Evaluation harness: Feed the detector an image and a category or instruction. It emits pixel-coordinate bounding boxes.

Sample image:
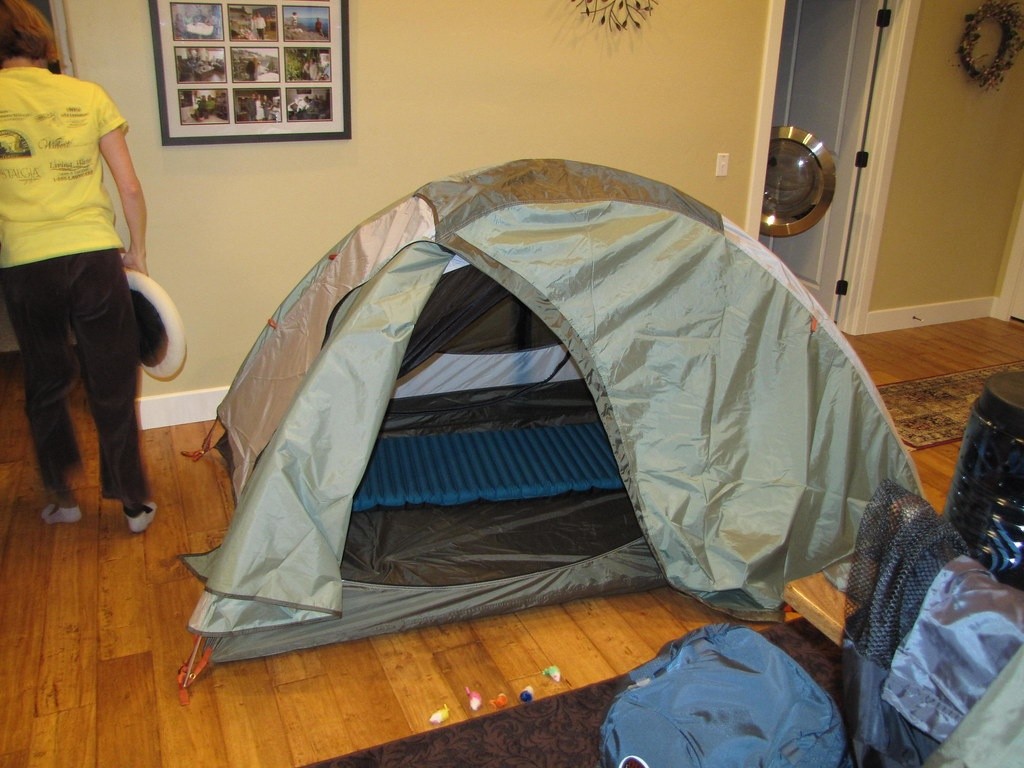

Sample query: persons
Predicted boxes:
[0,0,157,533]
[172,3,331,126]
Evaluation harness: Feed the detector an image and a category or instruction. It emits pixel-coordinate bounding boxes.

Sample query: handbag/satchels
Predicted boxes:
[600,622,846,768]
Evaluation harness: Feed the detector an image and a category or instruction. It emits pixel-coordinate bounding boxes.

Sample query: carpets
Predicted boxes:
[295,615,843,768]
[877,359,1024,452]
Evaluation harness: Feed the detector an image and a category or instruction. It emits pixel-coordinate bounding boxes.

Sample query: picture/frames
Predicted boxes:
[148,0,351,146]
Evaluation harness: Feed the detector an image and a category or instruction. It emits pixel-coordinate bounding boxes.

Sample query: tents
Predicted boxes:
[178,154,926,688]
